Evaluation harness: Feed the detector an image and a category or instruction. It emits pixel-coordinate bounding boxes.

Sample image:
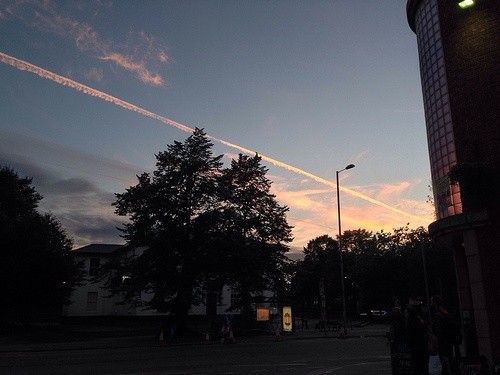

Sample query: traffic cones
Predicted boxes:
[219,323,237,345]
[272,324,284,342]
[158,328,167,347]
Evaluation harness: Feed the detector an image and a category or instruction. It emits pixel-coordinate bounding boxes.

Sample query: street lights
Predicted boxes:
[419,234,434,322]
[336,164,355,336]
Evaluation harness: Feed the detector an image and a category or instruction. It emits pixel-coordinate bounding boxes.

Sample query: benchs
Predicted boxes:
[314,322,342,332]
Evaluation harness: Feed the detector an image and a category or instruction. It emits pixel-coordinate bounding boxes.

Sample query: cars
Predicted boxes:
[359,308,390,322]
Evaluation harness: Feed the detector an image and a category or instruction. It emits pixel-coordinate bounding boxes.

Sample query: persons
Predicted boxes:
[446,294,463,365]
[220,308,237,344]
[438,191,451,211]
[430,294,454,375]
[383,306,432,375]
